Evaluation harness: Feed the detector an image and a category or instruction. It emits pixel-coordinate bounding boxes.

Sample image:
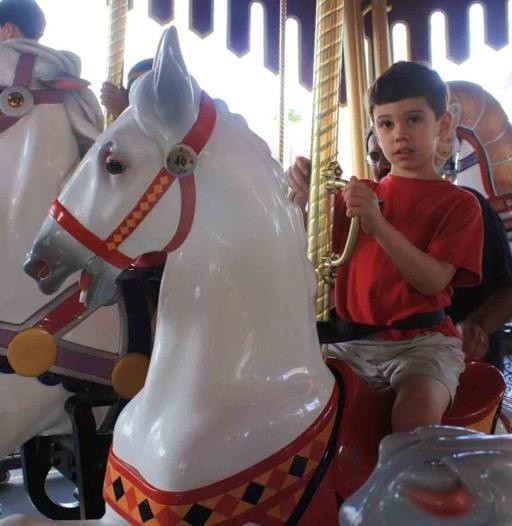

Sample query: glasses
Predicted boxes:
[366,152,381,165]
